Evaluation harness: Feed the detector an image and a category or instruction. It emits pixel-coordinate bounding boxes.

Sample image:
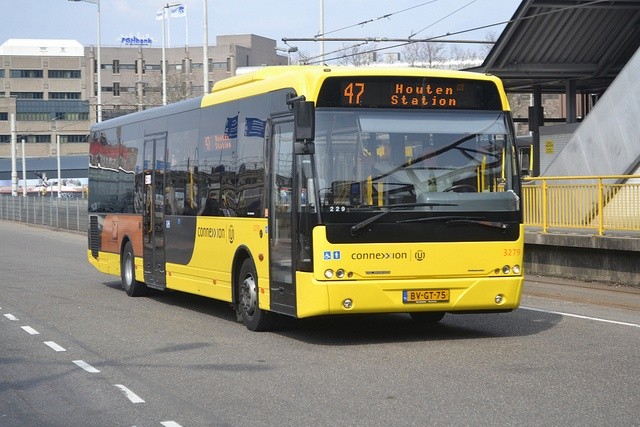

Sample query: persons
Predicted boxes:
[357,139,493,199]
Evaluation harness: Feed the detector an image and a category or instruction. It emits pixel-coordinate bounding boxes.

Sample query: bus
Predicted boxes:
[86,66,525,333]
